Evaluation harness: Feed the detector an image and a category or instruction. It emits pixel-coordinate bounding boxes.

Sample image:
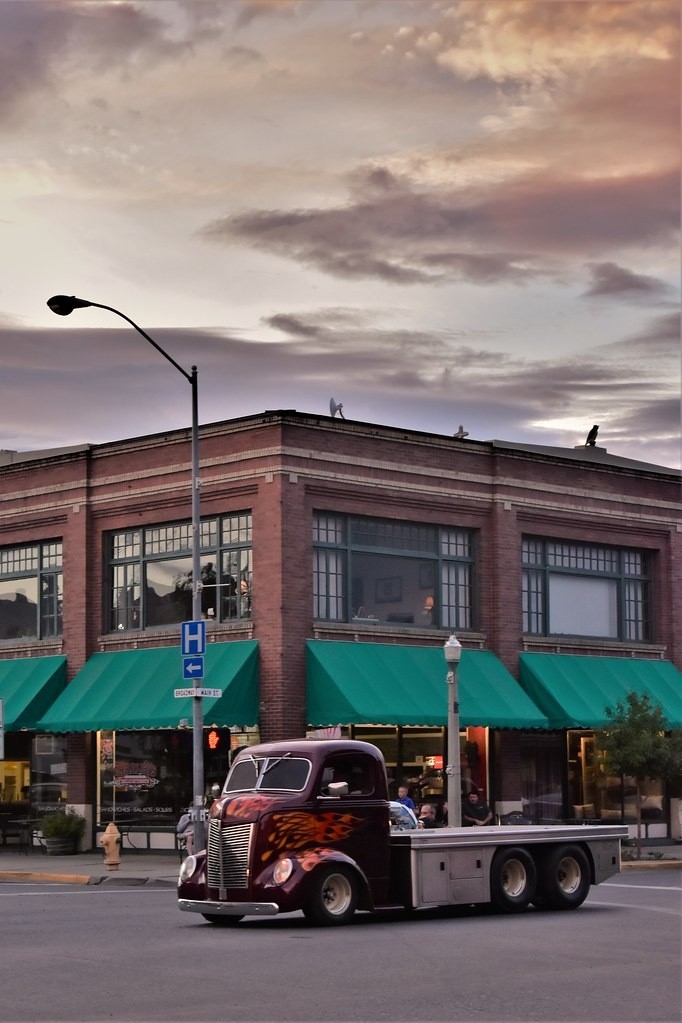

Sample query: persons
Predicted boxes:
[321,765,361,796]
[463,792,492,825]
[177,801,208,856]
[418,804,443,829]
[395,786,416,816]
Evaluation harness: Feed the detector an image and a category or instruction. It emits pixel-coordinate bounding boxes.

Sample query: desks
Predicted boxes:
[10,818,43,857]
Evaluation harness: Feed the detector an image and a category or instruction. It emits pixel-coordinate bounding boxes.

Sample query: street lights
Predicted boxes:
[443,634,463,826]
[47,293,206,854]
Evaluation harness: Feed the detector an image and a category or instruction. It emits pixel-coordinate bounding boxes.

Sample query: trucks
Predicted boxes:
[176,739,629,927]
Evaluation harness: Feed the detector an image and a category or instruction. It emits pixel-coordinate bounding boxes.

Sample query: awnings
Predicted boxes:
[518,651,682,730]
[37,639,260,729]
[305,639,549,728]
[0,654,67,732]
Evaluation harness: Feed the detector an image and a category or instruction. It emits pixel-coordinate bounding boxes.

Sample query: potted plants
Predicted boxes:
[40,808,86,856]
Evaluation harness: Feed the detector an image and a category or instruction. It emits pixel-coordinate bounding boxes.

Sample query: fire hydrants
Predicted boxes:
[100,822,122,871]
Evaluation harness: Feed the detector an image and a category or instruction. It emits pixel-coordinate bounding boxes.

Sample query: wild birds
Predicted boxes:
[585,425,599,446]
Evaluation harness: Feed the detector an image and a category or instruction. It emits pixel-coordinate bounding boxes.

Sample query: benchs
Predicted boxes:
[0,813,24,850]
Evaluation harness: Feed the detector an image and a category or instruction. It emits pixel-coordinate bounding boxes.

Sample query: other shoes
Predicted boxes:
[176,834,184,839]
